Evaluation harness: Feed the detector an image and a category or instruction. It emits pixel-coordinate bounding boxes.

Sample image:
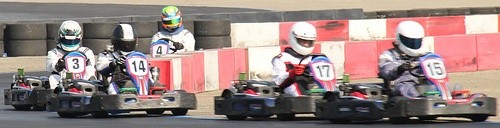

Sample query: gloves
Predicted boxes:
[288,66,305,78]
[399,63,413,75]
[109,55,126,69]
[55,58,66,71]
[173,42,184,51]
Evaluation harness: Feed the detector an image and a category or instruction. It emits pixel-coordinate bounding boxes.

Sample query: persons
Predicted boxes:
[96,24,170,103]
[149,5,195,56]
[45,20,96,98]
[271,21,370,113]
[377,21,480,108]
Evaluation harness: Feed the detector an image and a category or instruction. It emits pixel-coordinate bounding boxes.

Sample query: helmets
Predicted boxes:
[56,20,83,52]
[111,23,138,54]
[161,6,183,31]
[289,21,316,56]
[395,21,425,56]
